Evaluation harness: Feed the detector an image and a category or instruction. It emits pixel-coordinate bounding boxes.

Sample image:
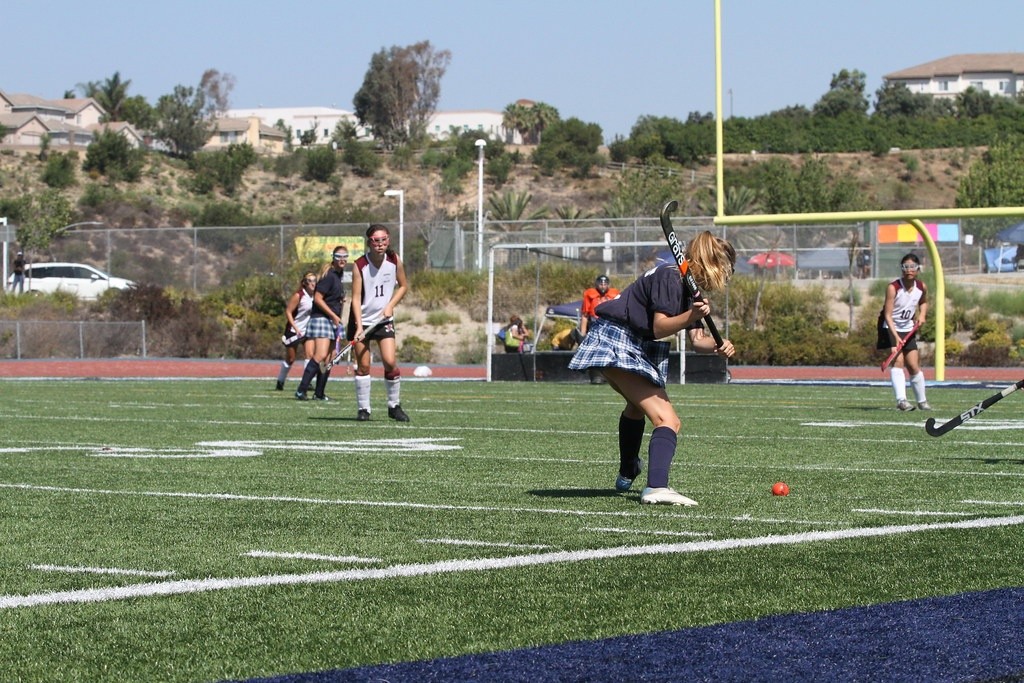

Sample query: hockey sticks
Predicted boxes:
[335,321,340,365]
[318,314,386,375]
[925,379,1024,438]
[280,331,306,346]
[661,200,724,348]
[881,321,919,372]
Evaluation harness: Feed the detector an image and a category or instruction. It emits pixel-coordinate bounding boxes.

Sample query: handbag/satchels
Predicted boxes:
[506,327,520,347]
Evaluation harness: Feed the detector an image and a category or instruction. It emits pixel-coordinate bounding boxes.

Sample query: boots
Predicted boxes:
[387,403,409,422]
[354,408,372,421]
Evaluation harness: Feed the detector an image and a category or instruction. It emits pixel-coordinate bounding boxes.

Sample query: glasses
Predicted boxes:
[369,236,389,246]
[304,278,317,284]
[334,253,349,261]
[900,263,920,272]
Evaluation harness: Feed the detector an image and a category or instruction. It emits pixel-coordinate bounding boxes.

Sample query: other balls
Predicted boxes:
[773,482,789,497]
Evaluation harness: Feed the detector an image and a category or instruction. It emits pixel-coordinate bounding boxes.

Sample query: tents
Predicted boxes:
[988,220,1024,276]
[531,300,585,354]
[747,251,796,281]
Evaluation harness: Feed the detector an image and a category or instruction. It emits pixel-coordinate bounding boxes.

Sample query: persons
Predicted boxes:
[551,328,579,351]
[276,262,338,391]
[567,231,736,507]
[581,275,622,385]
[10,251,26,293]
[295,246,349,402]
[347,225,410,422]
[856,242,872,280]
[877,254,932,411]
[504,315,529,352]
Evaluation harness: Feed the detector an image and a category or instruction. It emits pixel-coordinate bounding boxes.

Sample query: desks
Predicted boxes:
[491,350,728,384]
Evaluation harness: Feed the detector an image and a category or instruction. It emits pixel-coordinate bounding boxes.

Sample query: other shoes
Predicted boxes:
[294,390,311,401]
[276,380,285,391]
[313,394,332,401]
[307,383,316,391]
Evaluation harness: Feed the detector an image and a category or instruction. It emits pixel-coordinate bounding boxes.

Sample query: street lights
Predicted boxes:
[475,139,487,272]
[384,189,403,260]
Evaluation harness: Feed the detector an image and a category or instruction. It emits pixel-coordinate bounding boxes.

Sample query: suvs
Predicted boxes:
[7,262,137,302]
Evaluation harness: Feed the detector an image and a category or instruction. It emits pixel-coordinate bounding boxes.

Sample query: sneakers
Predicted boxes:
[616,457,643,492]
[640,486,698,507]
[917,401,932,411]
[896,400,915,412]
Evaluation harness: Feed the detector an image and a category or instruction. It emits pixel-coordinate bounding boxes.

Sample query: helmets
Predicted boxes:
[596,275,609,294]
